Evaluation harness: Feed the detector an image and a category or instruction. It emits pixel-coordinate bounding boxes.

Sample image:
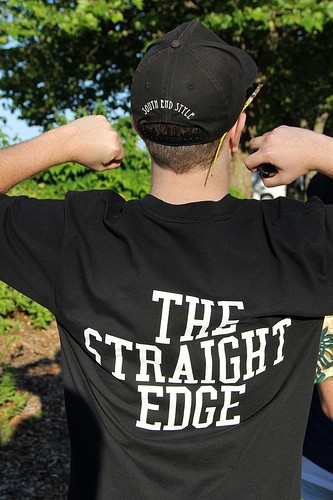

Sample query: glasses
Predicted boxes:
[204,83,265,188]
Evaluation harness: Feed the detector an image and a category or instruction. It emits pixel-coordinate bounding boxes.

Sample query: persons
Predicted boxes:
[302,315,333,499]
[0,20,333,500]
[251,127,307,202]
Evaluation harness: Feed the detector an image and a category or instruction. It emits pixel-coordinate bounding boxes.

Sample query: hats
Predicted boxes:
[131,20,257,146]
